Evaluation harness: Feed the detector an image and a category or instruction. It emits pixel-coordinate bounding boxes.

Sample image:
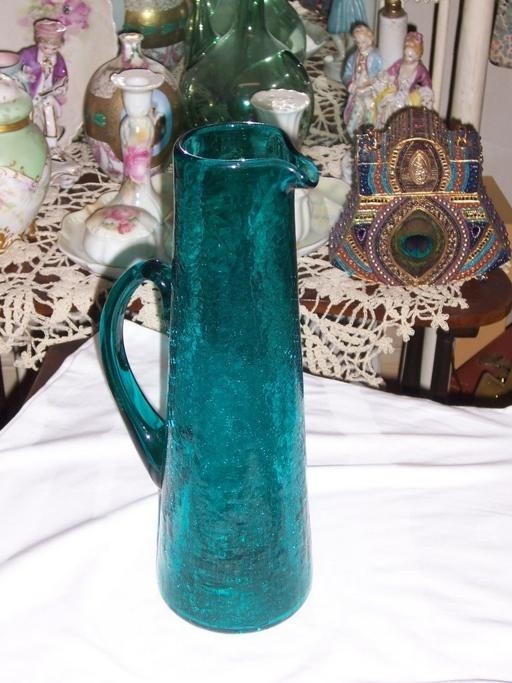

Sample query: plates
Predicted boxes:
[56,168,355,279]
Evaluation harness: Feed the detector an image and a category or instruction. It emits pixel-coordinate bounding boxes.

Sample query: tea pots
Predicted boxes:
[97,123,322,634]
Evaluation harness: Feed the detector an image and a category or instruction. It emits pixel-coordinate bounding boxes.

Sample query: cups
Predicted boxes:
[163,208,176,259]
